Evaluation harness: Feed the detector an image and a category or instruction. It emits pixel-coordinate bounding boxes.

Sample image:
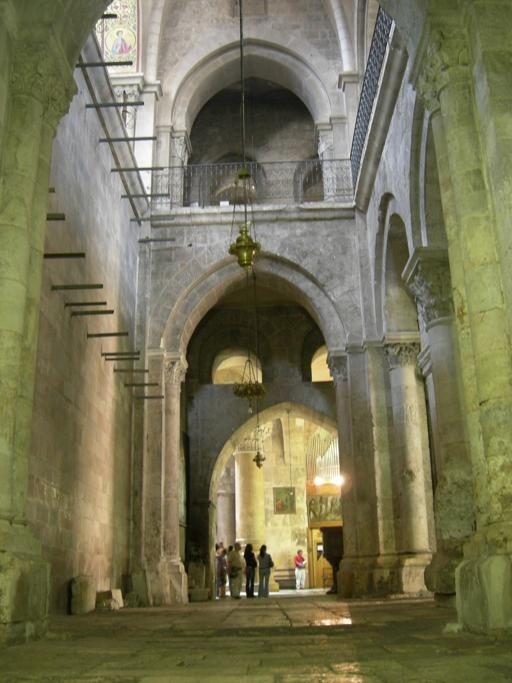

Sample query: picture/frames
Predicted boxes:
[272,485,296,515]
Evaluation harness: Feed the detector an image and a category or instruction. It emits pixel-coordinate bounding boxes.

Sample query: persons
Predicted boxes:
[295,549,306,590]
[244,544,257,598]
[256,544,271,598]
[216,541,243,599]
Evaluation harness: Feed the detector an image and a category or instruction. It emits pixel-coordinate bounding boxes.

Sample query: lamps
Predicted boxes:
[210,264,267,470]
[225,1,261,267]
